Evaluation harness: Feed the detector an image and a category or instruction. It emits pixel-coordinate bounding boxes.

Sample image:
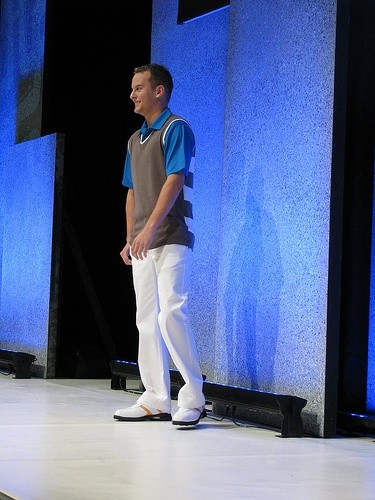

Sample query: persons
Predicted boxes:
[112,61,208,428]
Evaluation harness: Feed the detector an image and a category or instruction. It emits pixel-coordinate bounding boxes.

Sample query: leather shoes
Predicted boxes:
[113,402,172,424]
[171,403,207,427]
